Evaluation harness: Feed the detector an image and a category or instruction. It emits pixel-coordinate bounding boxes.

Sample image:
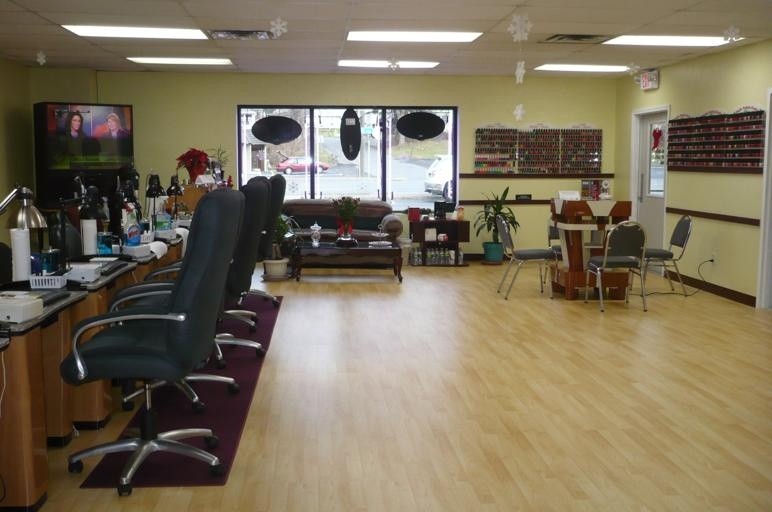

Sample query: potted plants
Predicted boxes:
[264,217,291,280]
[474,189,518,262]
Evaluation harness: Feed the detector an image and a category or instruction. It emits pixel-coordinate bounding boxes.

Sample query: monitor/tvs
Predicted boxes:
[33,102,135,174]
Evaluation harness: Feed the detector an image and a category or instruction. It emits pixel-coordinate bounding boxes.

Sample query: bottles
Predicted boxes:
[474,124,600,174]
[41,246,62,275]
[426,248,455,264]
[458,247,464,264]
[456,206,464,220]
[667,112,764,167]
[410,248,422,265]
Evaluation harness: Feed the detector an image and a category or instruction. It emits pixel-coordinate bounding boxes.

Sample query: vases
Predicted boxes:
[336,216,353,239]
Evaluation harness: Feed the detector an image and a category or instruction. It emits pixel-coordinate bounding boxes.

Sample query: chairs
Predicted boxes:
[496,209,689,313]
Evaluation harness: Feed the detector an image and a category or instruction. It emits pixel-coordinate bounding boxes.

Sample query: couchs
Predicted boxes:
[283,200,403,240]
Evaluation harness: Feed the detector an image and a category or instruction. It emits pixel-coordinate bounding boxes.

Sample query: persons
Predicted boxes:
[65,112,86,139]
[100,113,129,139]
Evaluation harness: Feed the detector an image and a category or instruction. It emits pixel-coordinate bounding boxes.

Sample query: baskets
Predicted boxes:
[28,270,71,289]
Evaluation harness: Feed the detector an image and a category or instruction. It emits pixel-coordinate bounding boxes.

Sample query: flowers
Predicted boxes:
[176,149,208,181]
[332,196,361,215]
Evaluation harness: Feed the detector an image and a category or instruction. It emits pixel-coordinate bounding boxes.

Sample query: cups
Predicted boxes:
[156,214,171,230]
[438,234,448,242]
[98,232,113,254]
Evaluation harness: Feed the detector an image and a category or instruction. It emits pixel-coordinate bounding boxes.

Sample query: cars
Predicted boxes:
[277,156,330,175]
[424,154,449,199]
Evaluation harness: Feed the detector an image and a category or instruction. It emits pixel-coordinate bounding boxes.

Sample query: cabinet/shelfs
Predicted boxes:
[669,110,764,173]
[459,127,614,178]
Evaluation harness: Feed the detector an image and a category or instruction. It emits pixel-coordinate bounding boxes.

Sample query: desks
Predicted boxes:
[411,219,470,266]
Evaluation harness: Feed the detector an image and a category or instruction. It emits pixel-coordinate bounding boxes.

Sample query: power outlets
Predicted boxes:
[709,255,719,265]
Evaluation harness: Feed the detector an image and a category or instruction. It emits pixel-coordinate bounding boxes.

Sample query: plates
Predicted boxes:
[369,241,392,247]
[372,233,390,237]
